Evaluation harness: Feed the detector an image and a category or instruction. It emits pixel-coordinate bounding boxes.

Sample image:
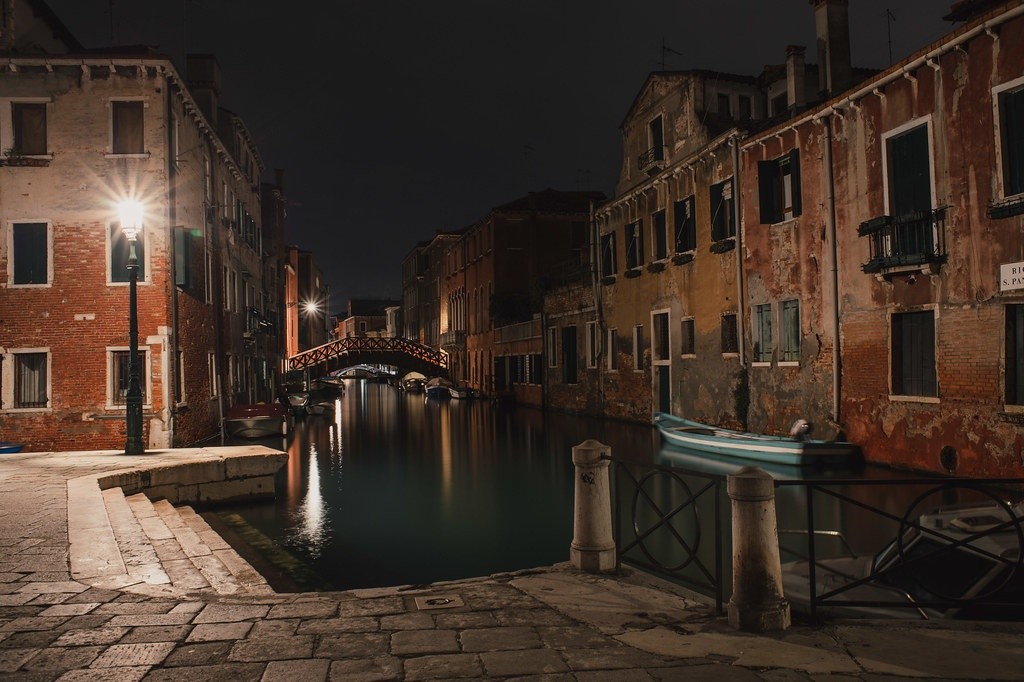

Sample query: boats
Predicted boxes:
[224,391,310,440]
[658,443,857,499]
[399,371,474,399]
[653,412,855,465]
[777,499,1024,620]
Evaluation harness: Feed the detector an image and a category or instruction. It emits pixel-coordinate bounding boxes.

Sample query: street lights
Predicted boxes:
[115,196,147,453]
